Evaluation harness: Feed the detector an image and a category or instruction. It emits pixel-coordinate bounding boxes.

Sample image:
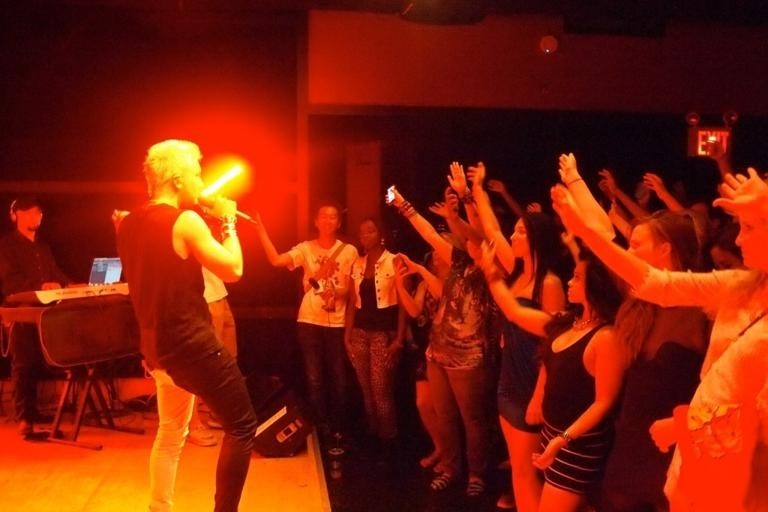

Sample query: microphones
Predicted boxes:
[308,276,336,311]
[195,192,248,221]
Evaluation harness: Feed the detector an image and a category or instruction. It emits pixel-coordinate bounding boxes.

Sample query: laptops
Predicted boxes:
[86,255,123,283]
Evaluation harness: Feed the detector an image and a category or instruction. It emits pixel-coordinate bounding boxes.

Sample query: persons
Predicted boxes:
[255,139,767,511]
[1,195,73,440]
[115,140,256,512]
[187,267,238,446]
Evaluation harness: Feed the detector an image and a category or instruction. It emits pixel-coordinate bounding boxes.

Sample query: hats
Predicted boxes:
[9,194,48,211]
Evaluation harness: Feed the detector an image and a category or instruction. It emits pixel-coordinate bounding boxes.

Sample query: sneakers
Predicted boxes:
[359,428,487,497]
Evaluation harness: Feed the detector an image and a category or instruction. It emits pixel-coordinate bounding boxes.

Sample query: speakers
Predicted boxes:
[243,367,313,459]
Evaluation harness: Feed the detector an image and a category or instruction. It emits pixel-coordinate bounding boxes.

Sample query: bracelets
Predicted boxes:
[220,217,238,239]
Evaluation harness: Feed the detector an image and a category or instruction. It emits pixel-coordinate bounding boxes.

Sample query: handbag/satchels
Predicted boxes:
[252,363,310,457]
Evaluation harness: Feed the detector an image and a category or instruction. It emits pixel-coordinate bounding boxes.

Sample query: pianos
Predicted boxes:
[5,282,129,305]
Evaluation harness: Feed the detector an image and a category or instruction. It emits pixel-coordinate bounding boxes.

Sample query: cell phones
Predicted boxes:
[388,185,395,204]
